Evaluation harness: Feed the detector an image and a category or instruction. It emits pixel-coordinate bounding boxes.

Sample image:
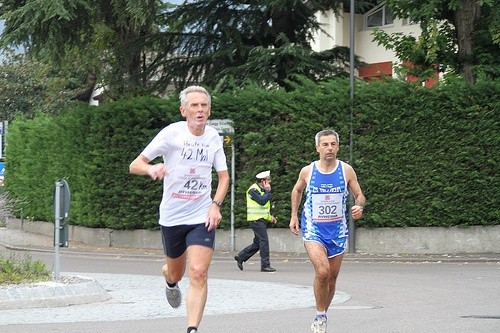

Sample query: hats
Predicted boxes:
[255,170,272,180]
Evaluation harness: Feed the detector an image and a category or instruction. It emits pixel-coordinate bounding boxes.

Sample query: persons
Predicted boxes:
[235,170,276,272]
[289,129,366,333]
[129,84,230,333]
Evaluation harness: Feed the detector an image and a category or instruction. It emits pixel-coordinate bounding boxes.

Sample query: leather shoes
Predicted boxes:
[261,267,276,272]
[234,255,243,270]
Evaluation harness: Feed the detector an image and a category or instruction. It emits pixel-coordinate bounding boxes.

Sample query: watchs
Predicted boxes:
[212,199,222,207]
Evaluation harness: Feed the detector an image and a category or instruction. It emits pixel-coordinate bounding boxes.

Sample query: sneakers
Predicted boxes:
[166,282,182,308]
[311,314,328,333]
